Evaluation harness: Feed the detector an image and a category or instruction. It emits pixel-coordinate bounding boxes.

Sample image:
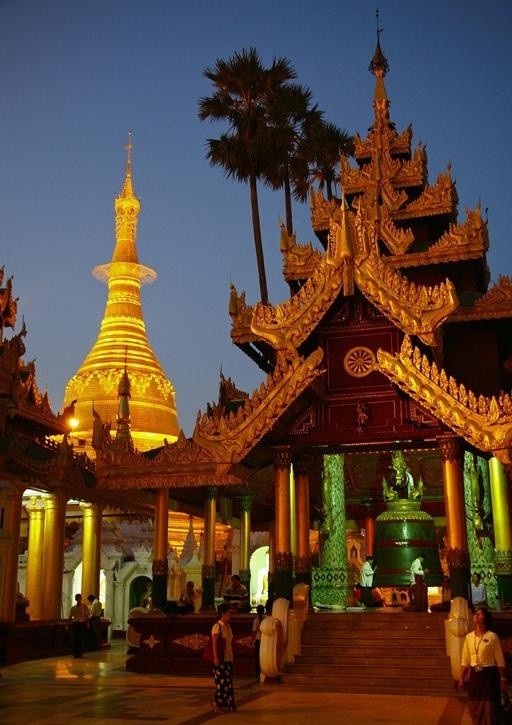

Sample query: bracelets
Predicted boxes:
[501,677,508,682]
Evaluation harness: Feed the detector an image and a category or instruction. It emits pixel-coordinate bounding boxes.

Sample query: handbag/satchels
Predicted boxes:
[202,622,225,665]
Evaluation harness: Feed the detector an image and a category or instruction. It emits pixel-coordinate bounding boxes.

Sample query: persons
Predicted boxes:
[456,608,509,725]
[66,594,89,654]
[222,574,252,613]
[15,593,30,624]
[472,572,488,611]
[251,605,268,680]
[357,556,379,608]
[88,592,102,648]
[402,574,429,612]
[172,582,199,616]
[409,553,429,600]
[211,603,246,714]
